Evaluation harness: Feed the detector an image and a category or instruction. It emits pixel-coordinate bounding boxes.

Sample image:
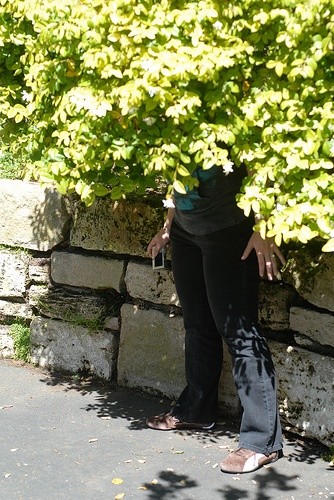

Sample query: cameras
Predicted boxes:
[152,246,164,269]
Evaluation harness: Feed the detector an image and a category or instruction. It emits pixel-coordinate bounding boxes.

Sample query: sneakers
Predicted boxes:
[146,409,216,431]
[220,447,279,473]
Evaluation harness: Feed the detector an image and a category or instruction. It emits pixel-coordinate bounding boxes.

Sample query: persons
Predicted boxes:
[146,140,289,473]
